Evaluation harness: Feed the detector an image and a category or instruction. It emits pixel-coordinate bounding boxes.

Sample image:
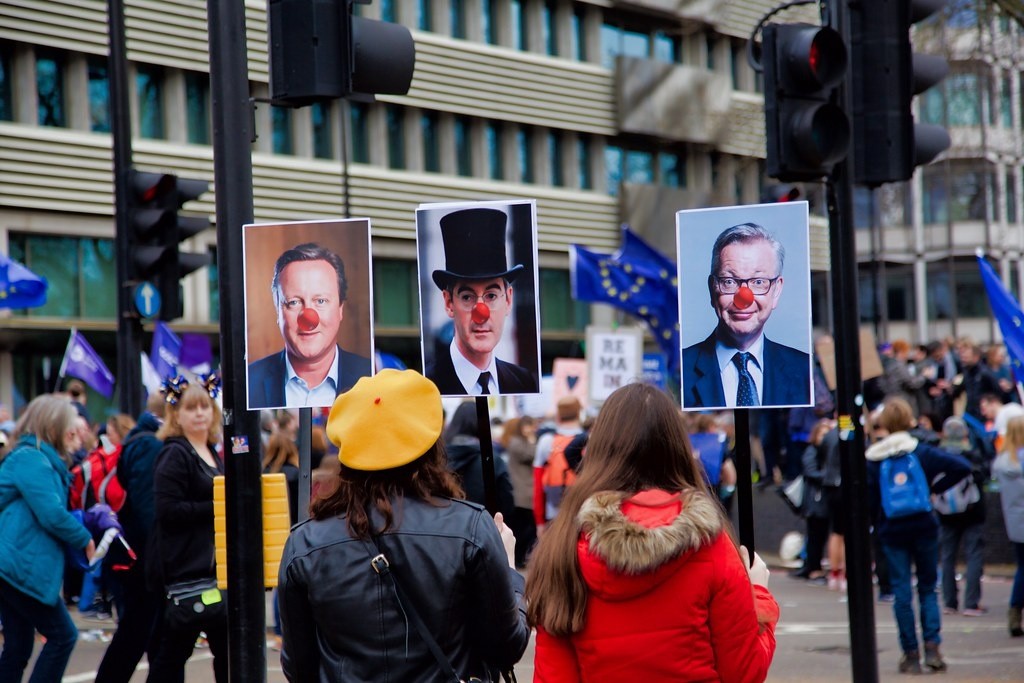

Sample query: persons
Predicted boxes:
[446,397,595,572]
[866,336,1024,676]
[277,369,532,683]
[526,382,781,683]
[682,409,846,593]
[249,242,372,408]
[426,208,536,395]
[0,382,226,683]
[260,410,339,650]
[683,222,810,408]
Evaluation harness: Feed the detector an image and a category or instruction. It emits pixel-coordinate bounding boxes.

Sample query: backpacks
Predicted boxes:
[876,442,934,516]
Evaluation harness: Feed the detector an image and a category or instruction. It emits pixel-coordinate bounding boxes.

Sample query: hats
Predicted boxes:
[326,367,445,468]
[943,417,969,438]
[557,398,580,418]
[432,206,524,290]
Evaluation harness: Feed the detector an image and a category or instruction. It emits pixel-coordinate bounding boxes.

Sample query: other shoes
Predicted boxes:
[876,594,895,605]
[828,569,846,590]
[964,606,990,616]
[943,605,958,616]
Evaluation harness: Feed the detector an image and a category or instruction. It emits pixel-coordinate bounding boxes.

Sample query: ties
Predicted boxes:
[478,371,491,395]
[732,351,759,406]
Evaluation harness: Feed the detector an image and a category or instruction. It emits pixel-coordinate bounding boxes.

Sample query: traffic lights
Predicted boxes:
[266,1,415,105]
[129,171,212,321]
[823,0,953,191]
[763,21,850,182]
[766,187,798,208]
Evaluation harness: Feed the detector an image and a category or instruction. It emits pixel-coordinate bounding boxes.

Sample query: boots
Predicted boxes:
[1007,608,1023,636]
[925,641,946,671]
[899,650,919,673]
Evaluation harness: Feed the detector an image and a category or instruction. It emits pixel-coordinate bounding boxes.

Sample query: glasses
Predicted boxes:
[714,273,779,295]
[450,289,508,311]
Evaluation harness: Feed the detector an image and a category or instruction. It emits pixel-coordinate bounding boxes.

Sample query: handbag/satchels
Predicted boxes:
[461,657,517,683]
[783,475,805,515]
[169,578,221,627]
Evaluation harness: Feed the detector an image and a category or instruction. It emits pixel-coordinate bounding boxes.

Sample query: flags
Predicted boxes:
[64,335,115,401]
[977,256,1024,403]
[572,227,679,376]
[0,253,49,309]
